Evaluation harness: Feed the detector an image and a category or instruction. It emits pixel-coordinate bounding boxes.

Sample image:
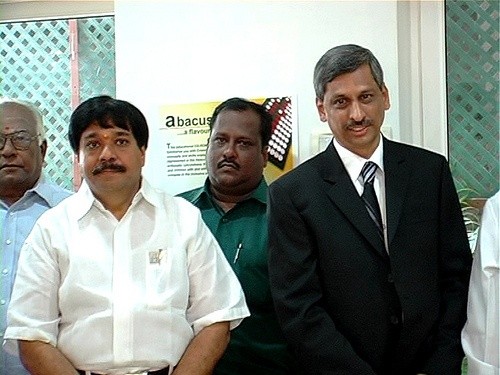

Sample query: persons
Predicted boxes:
[265,43,474,375]
[166,93,276,375]
[0,101,76,373]
[6,93,251,375]
[461,191,499,375]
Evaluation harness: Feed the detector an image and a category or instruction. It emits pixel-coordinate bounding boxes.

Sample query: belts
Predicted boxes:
[75,365,169,375]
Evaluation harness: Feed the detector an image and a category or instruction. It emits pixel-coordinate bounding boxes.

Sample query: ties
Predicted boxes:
[361,161,385,247]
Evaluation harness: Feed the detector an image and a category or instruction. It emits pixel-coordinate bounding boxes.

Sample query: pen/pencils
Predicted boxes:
[231,241,244,265]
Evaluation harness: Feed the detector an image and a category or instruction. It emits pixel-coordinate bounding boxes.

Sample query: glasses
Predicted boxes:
[0,129,38,151]
